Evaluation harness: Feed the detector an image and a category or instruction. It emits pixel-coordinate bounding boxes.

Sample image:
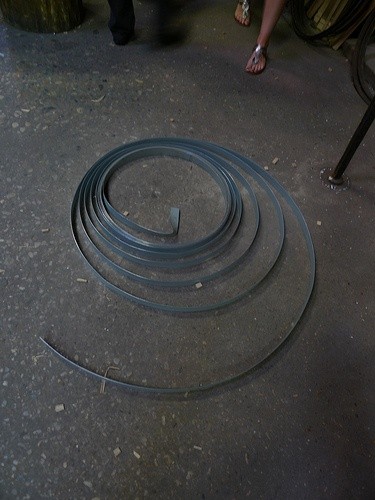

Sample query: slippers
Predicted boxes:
[235,0,251,26]
[249,43,266,74]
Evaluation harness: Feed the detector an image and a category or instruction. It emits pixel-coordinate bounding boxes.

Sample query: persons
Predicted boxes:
[107,0,189,48]
[234,0,284,75]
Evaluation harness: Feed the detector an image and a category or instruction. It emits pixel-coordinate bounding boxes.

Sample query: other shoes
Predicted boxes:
[114,30,137,46]
[154,30,187,49]
